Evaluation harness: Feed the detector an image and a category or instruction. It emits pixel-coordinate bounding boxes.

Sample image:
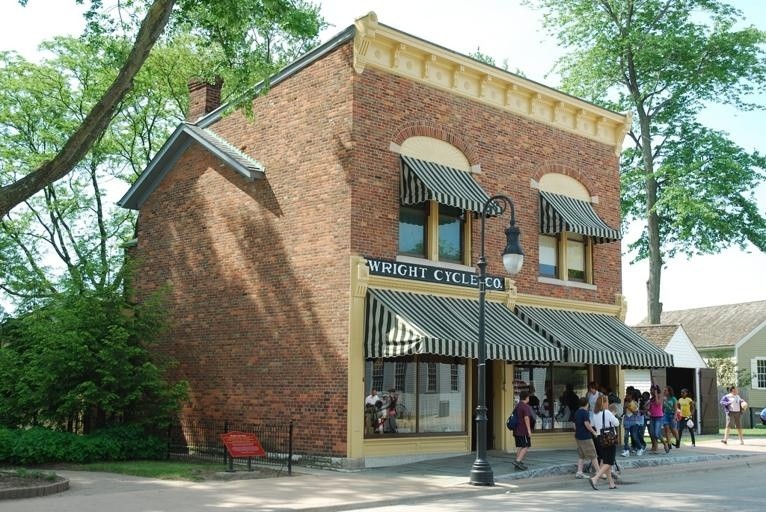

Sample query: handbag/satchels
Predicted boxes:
[506,412,520,431]
[600,427,619,448]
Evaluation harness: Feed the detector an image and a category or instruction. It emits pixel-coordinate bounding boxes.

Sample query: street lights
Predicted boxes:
[469,193,525,488]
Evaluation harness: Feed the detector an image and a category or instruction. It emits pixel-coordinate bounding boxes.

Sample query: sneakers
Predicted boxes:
[620,446,648,458]
[609,480,617,488]
[589,477,599,490]
[512,460,525,471]
[575,472,590,479]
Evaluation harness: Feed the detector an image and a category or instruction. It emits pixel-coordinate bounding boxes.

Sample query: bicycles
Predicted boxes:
[371,388,411,422]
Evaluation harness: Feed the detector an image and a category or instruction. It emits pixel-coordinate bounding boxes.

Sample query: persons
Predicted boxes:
[527,383,580,430]
[720,384,747,444]
[571,380,696,491]
[364,385,399,433]
[512,390,535,471]
